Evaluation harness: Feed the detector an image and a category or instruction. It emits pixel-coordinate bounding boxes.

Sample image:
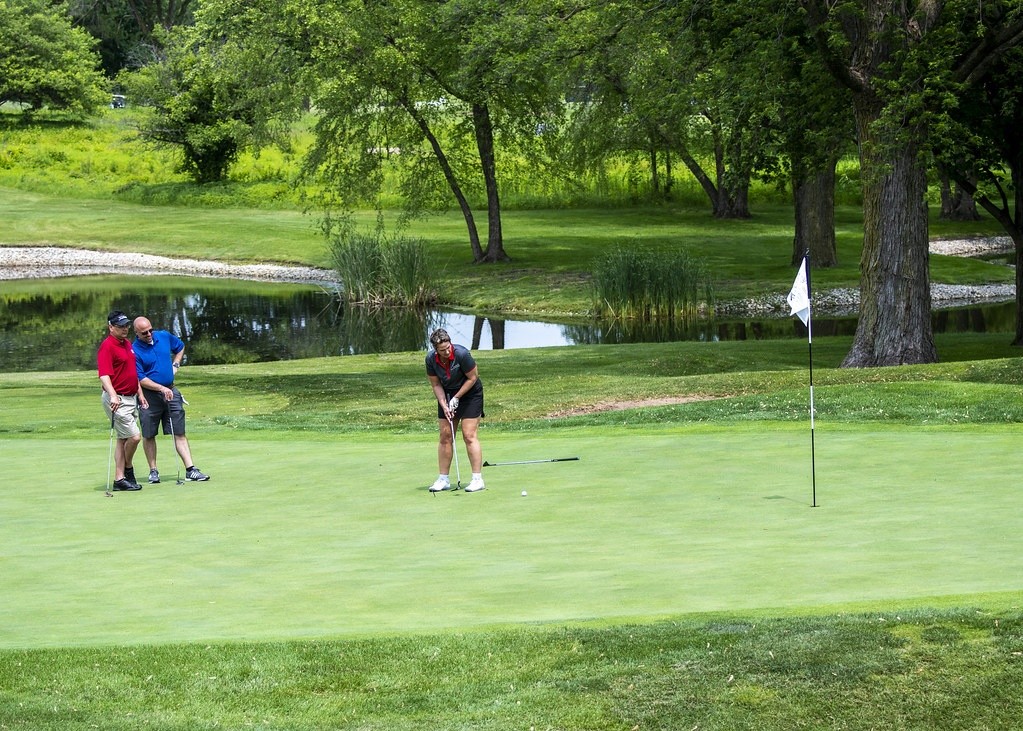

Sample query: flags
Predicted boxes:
[786,250,812,326]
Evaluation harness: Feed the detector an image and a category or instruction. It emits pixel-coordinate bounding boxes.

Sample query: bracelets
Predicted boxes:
[173,362,179,368]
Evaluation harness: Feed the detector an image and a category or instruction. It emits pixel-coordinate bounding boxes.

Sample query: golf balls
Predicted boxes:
[521,490,527,497]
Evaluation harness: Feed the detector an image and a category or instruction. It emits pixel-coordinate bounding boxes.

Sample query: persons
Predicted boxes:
[99,311,210,490]
[425,328,486,493]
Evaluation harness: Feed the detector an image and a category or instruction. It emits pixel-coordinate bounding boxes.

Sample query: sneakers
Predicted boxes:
[429,478,450,492]
[124,466,136,484]
[185,467,210,481]
[113,478,142,491]
[465,478,485,492]
[148,470,160,483]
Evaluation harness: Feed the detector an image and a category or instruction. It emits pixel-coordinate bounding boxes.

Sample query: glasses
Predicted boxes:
[138,328,154,335]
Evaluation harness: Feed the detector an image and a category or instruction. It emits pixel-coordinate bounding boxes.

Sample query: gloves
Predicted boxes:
[449,397,459,411]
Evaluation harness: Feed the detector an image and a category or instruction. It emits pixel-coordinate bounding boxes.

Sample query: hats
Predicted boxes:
[108,313,133,326]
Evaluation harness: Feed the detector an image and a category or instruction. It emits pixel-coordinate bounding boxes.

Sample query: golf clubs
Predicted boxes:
[165,394,186,486]
[482,456,580,468]
[104,405,116,498]
[446,393,462,492]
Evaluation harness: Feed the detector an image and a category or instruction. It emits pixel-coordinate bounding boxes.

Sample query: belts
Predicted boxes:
[142,383,174,393]
[117,392,134,396]
